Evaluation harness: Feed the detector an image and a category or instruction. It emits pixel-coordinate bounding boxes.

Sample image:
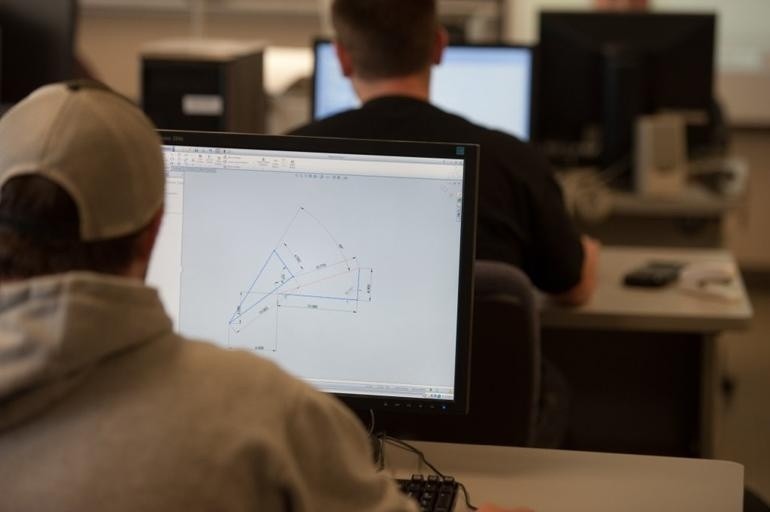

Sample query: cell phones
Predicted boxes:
[624,261,679,289]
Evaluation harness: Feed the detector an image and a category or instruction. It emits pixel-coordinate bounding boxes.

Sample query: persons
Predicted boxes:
[0,75,535,512]
[266,1,611,363]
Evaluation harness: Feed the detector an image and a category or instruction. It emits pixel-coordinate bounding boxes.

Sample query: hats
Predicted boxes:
[0,80,167,244]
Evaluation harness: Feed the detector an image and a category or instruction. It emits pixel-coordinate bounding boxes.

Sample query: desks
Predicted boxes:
[577,190,733,246]
[382,437,746,511]
[531,246,756,432]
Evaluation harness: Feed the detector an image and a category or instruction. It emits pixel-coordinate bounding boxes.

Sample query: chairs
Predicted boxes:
[384,257,544,443]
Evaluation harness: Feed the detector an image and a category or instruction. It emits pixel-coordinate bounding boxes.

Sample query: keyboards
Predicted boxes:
[385,470,459,512]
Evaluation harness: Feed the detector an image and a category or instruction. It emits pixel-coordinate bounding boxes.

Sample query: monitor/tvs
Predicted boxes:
[537,9,719,204]
[144,124,481,468]
[312,38,533,142]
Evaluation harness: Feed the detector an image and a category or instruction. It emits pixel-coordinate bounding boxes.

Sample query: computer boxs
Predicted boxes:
[136,41,265,131]
